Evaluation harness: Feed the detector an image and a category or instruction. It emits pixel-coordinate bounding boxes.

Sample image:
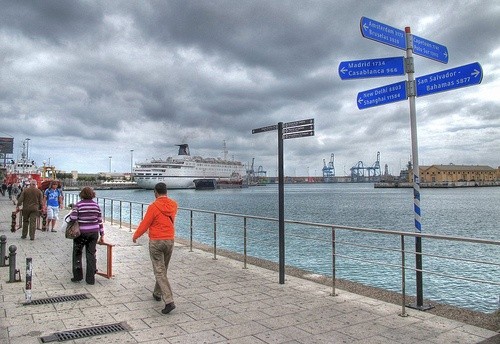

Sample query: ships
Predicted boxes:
[134,139,247,190]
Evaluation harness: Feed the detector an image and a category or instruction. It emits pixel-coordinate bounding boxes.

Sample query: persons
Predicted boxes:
[133,183,178,314]
[71,186,105,285]
[0,181,64,240]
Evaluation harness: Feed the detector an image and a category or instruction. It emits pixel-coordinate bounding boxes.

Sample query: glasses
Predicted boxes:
[54,184,57,185]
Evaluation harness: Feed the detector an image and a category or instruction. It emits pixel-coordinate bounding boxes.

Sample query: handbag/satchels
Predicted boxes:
[65,214,81,238]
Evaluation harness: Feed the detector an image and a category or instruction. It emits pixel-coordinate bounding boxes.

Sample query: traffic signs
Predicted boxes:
[357,79,408,109]
[338,56,407,80]
[360,16,408,50]
[282,118,314,139]
[251,125,278,134]
[413,34,449,65]
[415,62,483,97]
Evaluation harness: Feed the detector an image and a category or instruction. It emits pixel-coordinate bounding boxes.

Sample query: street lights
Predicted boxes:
[130,149,134,182]
[25,138,31,162]
[108,156,112,173]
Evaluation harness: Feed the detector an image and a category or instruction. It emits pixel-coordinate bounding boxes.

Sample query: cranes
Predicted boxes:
[350,151,382,177]
[321,153,335,178]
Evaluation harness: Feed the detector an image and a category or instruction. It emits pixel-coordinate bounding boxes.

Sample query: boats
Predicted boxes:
[101,179,137,190]
[5,140,63,197]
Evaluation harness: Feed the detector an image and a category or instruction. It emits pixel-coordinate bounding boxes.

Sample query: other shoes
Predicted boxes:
[161,303,176,314]
[71,278,81,283]
[21,236,26,239]
[30,237,34,240]
[153,293,161,301]
[51,229,57,232]
